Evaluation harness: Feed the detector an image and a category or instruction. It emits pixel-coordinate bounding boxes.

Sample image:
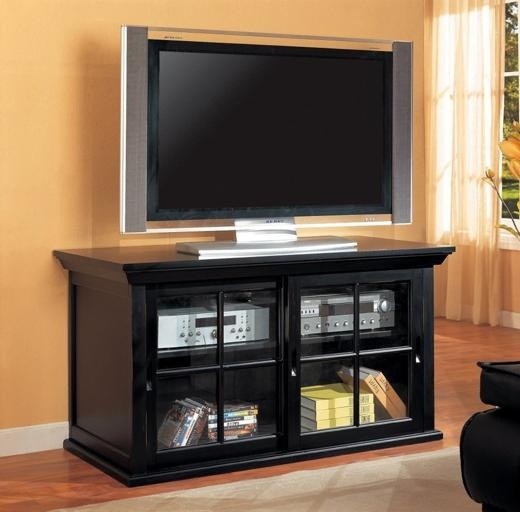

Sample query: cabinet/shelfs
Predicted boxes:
[51,234,456,488]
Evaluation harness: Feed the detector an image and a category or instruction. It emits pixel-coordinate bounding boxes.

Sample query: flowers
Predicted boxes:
[481,110,519,248]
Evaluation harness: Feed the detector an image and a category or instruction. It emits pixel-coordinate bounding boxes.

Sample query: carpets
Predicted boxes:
[69,446,483,511]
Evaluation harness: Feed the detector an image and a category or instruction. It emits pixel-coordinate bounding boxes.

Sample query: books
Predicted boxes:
[297,406,375,421]
[296,417,374,430]
[360,366,407,416]
[336,367,404,419]
[299,384,374,412]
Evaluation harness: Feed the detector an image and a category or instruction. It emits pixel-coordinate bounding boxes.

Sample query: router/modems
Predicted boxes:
[156,302,271,350]
[301,288,396,336]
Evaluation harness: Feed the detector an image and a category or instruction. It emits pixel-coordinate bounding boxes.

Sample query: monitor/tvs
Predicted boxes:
[119,23,414,261]
